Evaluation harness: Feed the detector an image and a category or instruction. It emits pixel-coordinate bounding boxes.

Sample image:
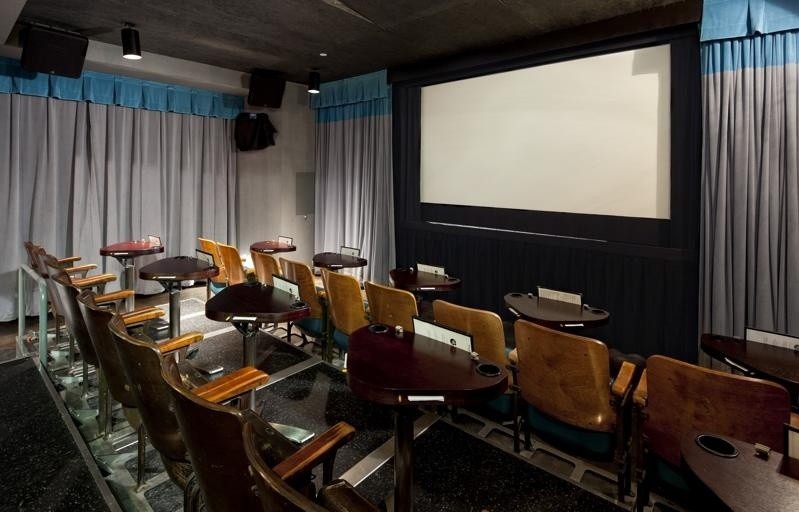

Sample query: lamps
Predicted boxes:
[302,66,324,96]
[121,19,144,61]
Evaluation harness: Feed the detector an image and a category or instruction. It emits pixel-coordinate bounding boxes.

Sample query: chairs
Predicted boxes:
[45,261,116,395]
[364,279,424,334]
[217,243,249,288]
[107,314,270,512]
[23,239,98,372]
[160,353,318,512]
[74,290,204,437]
[510,315,636,504]
[196,239,232,303]
[636,358,790,511]
[36,252,97,367]
[50,272,135,400]
[433,297,522,451]
[240,421,376,512]
[700,333,797,392]
[279,256,328,358]
[252,249,281,289]
[322,265,369,362]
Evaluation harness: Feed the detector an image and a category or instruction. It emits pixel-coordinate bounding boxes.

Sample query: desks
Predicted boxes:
[99,238,163,312]
[205,280,313,412]
[347,320,508,511]
[680,426,799,509]
[138,256,220,337]
[312,251,369,271]
[390,265,461,315]
[251,239,297,258]
[505,286,610,328]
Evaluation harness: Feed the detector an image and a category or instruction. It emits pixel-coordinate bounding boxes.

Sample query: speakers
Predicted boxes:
[248,69,286,108]
[20,24,90,79]
